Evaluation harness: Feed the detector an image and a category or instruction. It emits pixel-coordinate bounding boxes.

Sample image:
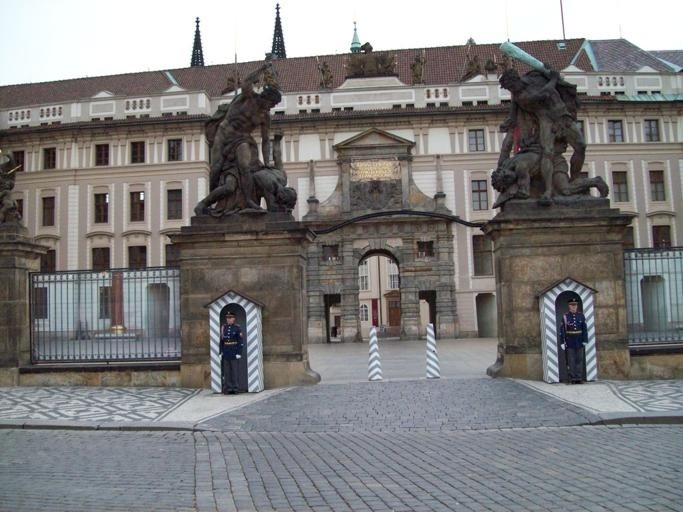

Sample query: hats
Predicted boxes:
[224,311,236,319]
[567,298,579,305]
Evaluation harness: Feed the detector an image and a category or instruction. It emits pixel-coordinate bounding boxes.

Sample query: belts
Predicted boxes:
[565,329,583,334]
[222,341,238,345]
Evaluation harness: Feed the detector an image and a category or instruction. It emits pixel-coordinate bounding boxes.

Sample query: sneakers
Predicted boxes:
[223,390,238,395]
[571,379,584,385]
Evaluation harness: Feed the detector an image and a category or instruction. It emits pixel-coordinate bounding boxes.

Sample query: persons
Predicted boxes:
[495,55,515,69]
[410,52,428,86]
[194,54,297,218]
[318,61,335,88]
[559,298,589,384]
[491,62,609,207]
[219,311,243,395]
[461,55,480,81]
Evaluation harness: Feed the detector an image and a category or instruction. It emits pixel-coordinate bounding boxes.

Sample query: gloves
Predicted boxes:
[236,354,242,360]
[218,352,224,361]
[581,342,588,347]
[560,343,566,351]
